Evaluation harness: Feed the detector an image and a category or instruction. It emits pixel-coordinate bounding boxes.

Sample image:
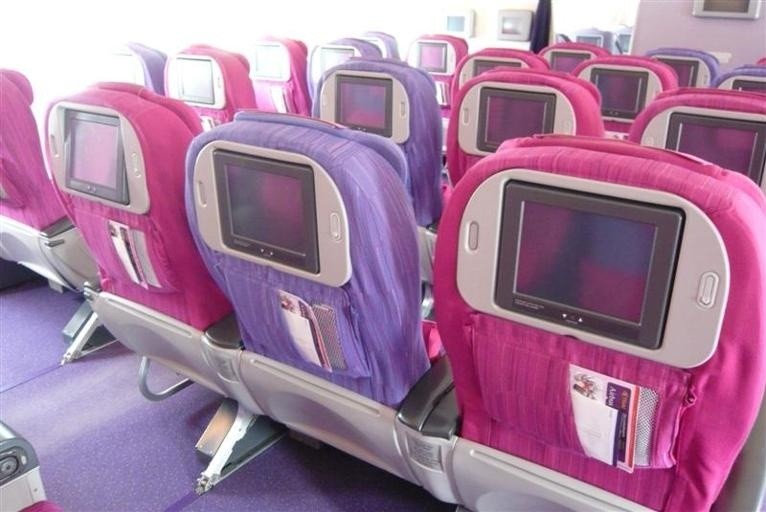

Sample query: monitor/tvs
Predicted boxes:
[170,56,217,107]
[211,150,322,275]
[334,73,394,138]
[61,108,132,204]
[317,46,354,74]
[656,57,700,90]
[500,15,523,33]
[476,87,556,152]
[416,41,447,73]
[79,117,86,121]
[665,108,766,188]
[252,42,290,79]
[446,15,463,31]
[732,80,766,93]
[495,181,683,349]
[473,59,522,74]
[577,35,597,44]
[551,51,590,76]
[590,67,649,125]
[91,56,146,84]
[617,33,628,49]
